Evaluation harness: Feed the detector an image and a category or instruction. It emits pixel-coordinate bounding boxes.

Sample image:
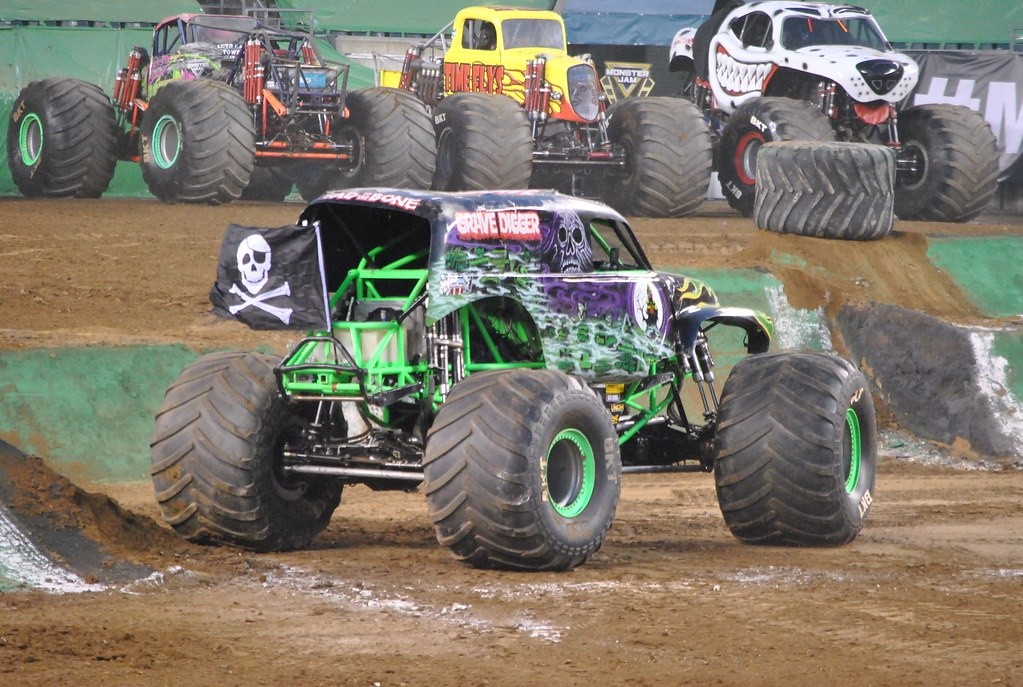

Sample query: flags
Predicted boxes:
[210,223,328,331]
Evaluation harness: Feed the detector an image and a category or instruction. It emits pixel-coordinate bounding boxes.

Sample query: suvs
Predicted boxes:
[147,186,879,572]
[666,0,998,225]
[5,12,437,206]
[397,4,713,219]
